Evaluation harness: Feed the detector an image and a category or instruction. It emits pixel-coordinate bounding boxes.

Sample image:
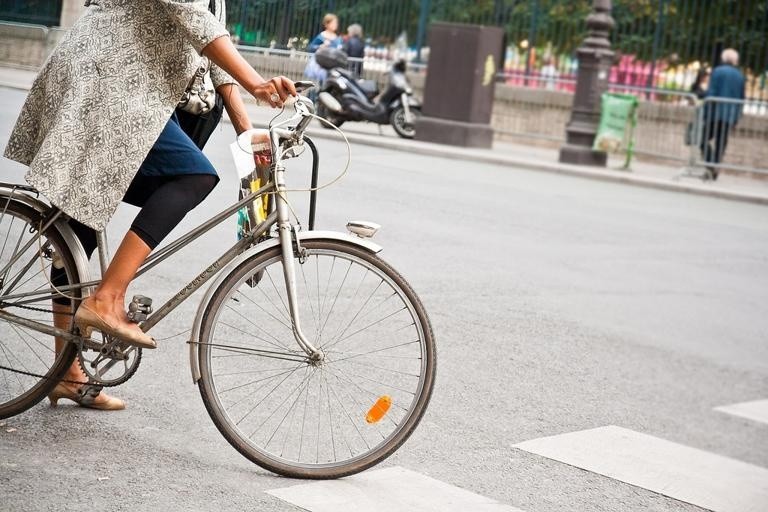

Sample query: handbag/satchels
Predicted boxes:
[230,128,279,288]
[179,54,218,116]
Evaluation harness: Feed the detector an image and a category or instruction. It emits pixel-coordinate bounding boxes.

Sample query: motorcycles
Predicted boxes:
[314,45,422,141]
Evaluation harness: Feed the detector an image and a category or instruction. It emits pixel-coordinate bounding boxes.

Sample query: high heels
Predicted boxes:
[74,300,157,349]
[47,380,127,411]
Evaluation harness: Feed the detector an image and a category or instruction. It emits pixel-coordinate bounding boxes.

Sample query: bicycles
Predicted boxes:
[0,80,435,477]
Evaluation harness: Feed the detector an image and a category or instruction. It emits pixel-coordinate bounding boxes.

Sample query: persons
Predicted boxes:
[539,55,559,92]
[302,13,343,116]
[694,49,746,182]
[3,1,298,409]
[684,62,712,147]
[338,23,364,78]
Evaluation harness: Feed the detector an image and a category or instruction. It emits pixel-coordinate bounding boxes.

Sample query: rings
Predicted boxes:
[271,93,279,103]
